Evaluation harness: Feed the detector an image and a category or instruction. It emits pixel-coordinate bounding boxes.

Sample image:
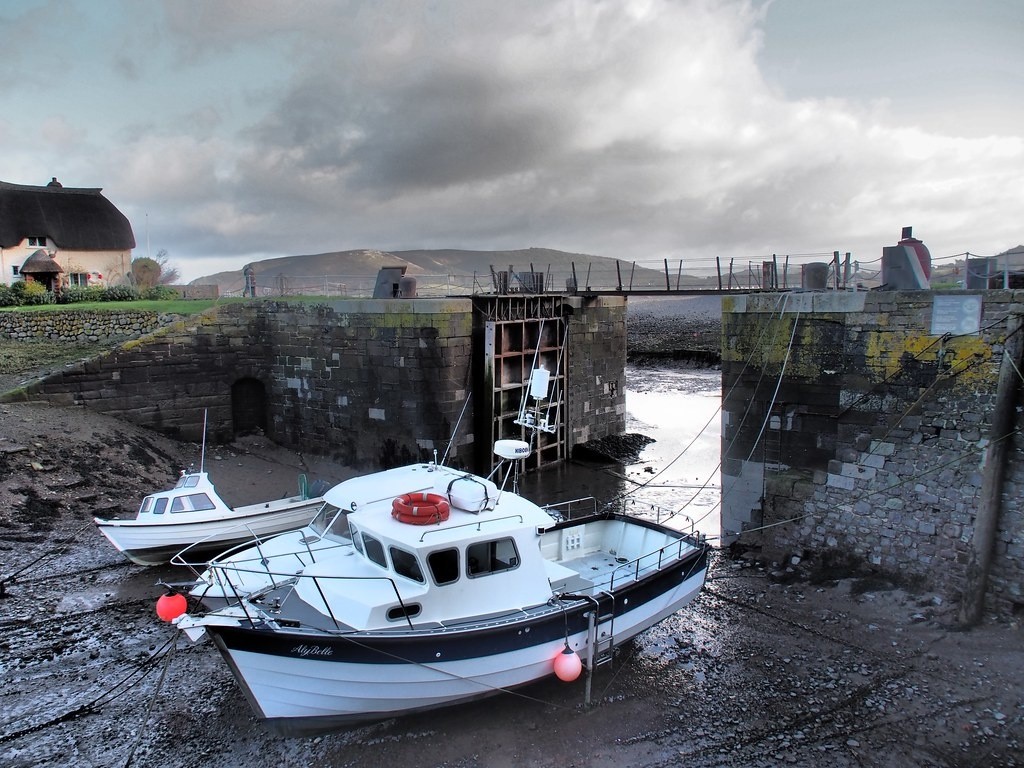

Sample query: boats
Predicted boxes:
[93,409,327,568]
[154,320,713,742]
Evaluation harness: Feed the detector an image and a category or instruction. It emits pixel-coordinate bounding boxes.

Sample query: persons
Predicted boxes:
[243,264,257,298]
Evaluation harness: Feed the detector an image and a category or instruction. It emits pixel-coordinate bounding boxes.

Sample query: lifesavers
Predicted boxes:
[391,489,450,526]
